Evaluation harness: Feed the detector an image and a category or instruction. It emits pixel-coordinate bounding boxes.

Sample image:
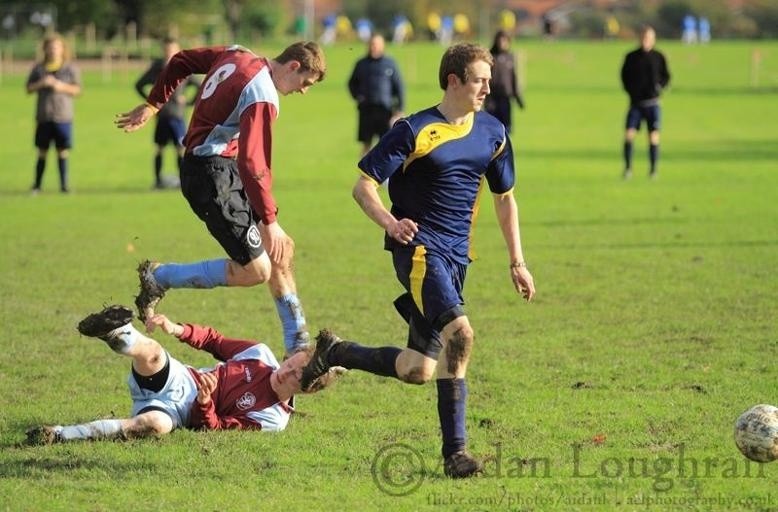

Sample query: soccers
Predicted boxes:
[734,405,778,462]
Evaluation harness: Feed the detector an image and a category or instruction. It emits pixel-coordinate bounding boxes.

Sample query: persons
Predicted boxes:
[25,34,82,194]
[133,35,202,189]
[114,40,330,356]
[345,32,407,158]
[300,41,537,479]
[26,304,335,447]
[485,29,523,134]
[619,25,672,182]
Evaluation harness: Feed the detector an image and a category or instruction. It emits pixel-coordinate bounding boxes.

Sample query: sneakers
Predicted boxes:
[301,333,339,393]
[28,424,60,446]
[444,451,483,477]
[77,305,136,338]
[135,261,160,324]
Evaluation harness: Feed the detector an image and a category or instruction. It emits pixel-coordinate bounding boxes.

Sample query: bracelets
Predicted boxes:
[144,101,160,115]
[510,257,529,269]
